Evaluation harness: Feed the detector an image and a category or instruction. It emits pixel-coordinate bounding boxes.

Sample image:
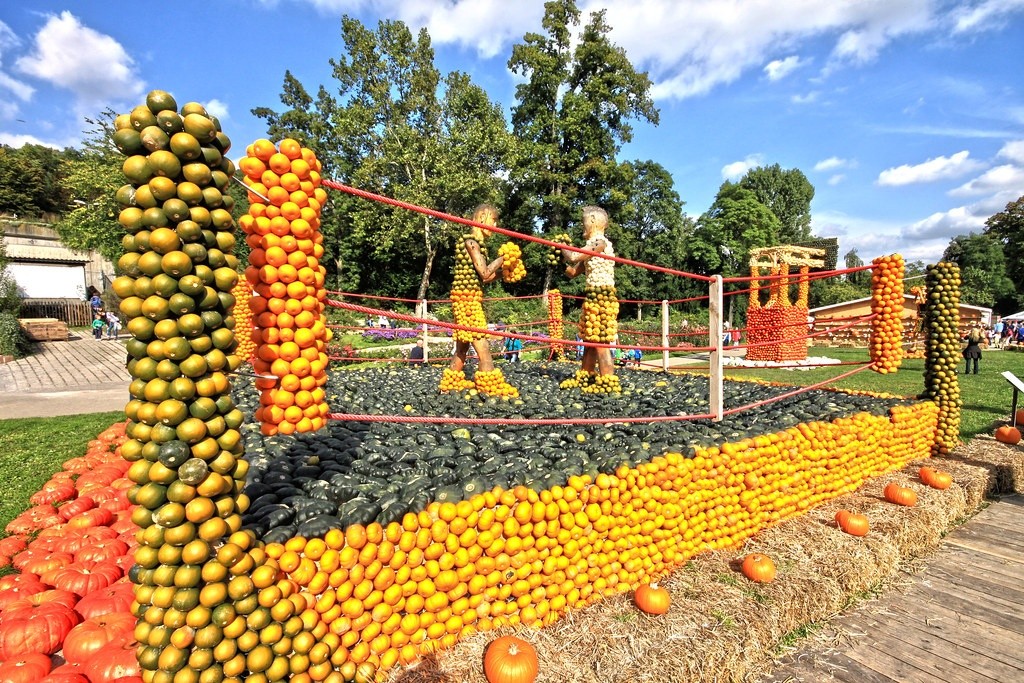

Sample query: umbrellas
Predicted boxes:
[1001,312,1024,319]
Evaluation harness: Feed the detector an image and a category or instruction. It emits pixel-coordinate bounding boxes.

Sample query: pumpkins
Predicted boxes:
[108,89,961,683]
[1016,407,1024,425]
[0,419,148,683]
[995,424,1021,445]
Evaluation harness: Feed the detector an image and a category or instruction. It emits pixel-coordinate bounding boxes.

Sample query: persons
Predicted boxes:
[575,333,643,367]
[365,308,396,329]
[409,340,428,366]
[438,204,526,395]
[501,330,521,363]
[722,320,741,346]
[962,320,1024,374]
[90,292,119,341]
[548,205,621,394]
